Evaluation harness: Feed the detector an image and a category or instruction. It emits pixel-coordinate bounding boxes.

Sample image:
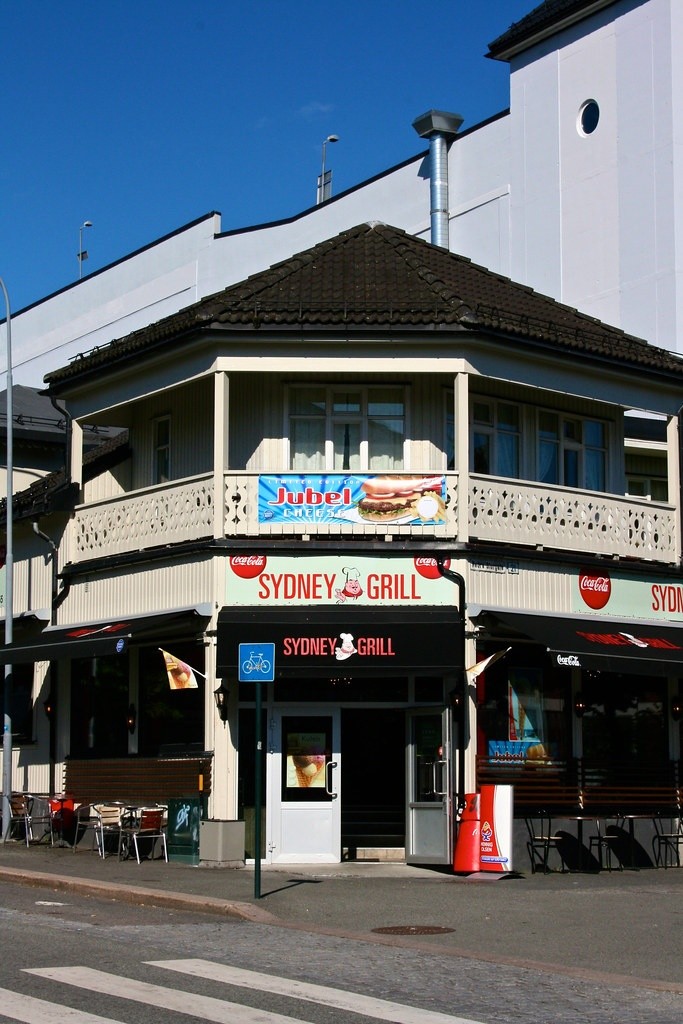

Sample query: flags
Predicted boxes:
[162,650,198,690]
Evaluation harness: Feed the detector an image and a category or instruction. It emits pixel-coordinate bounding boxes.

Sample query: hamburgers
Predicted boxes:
[358,475,444,522]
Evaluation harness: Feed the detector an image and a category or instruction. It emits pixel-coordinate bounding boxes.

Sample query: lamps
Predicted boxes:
[43,694,57,721]
[447,683,469,716]
[574,693,586,720]
[125,704,135,735]
[670,696,683,721]
[213,683,231,727]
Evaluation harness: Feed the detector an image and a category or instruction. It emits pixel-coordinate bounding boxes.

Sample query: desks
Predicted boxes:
[40,794,94,849]
[103,802,160,860]
[555,813,595,871]
[610,814,659,870]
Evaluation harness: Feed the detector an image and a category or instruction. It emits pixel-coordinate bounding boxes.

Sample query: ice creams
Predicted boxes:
[293,742,325,788]
[169,659,191,688]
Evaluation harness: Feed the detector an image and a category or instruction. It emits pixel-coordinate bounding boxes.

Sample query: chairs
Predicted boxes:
[2,792,203,866]
[521,808,567,875]
[589,811,626,872]
[648,807,683,867]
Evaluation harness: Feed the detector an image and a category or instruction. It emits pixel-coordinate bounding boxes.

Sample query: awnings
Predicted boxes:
[1,609,212,664]
[476,610,683,678]
[218,606,466,668]
